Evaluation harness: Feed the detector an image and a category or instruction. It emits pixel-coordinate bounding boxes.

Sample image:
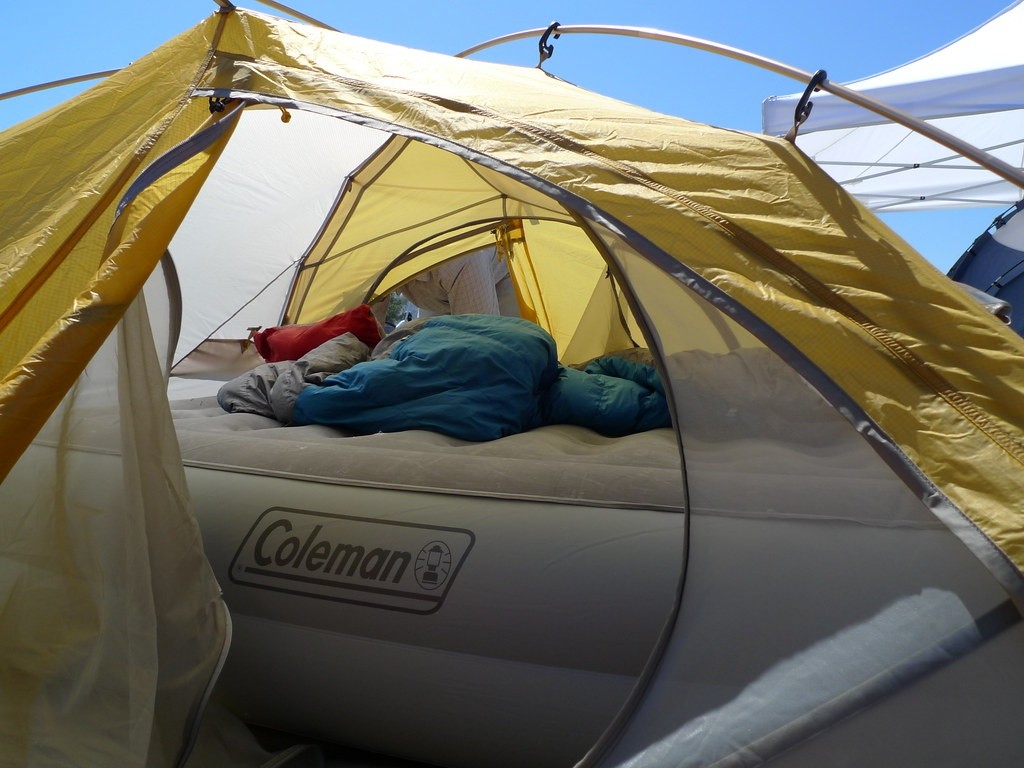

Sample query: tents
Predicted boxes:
[947,200,1024,340]
[761,0,1024,211]
[0,0,1023,768]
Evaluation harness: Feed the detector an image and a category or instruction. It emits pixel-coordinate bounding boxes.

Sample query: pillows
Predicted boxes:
[252,304,385,364]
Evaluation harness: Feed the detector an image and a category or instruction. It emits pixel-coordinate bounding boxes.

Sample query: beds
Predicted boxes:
[167,348,1024,768]
